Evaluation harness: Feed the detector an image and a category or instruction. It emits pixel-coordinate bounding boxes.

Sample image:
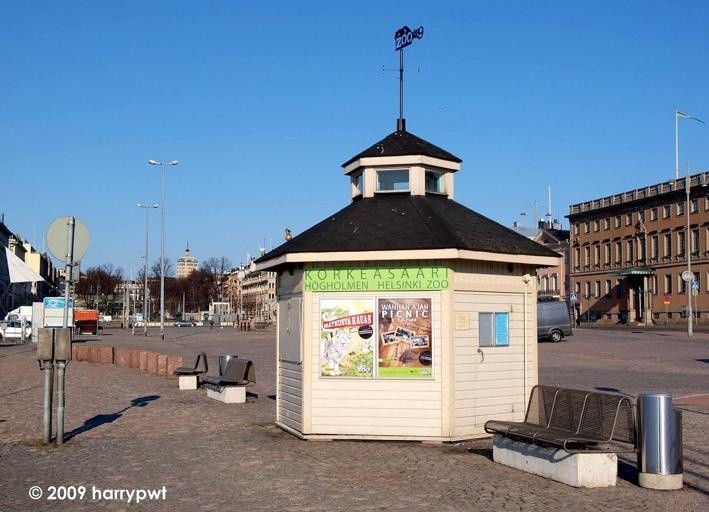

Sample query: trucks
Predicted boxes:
[3,306,77,328]
[72,307,99,336]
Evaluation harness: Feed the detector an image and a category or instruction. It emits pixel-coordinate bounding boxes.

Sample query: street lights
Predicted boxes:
[135,201,159,333]
[667,162,708,336]
[520,198,552,237]
[237,259,246,322]
[257,281,261,317]
[146,158,179,335]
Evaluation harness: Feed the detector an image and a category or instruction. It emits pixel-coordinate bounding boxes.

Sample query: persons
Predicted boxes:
[323,331,341,376]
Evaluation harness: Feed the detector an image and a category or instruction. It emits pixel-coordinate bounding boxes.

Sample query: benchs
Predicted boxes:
[173,353,208,390]
[484,384,640,489]
[202,356,257,403]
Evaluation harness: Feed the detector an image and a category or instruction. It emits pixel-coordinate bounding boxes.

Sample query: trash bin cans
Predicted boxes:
[219,355,238,375]
[637,391,683,490]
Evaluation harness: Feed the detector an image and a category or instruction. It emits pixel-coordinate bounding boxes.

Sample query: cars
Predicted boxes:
[0,320,32,342]
[173,318,196,328]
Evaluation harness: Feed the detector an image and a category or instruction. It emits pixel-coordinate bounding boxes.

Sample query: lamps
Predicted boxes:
[285,228,292,241]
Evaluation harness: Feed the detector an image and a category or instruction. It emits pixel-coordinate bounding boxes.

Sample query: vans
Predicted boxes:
[536,294,574,343]
[98,315,112,322]
